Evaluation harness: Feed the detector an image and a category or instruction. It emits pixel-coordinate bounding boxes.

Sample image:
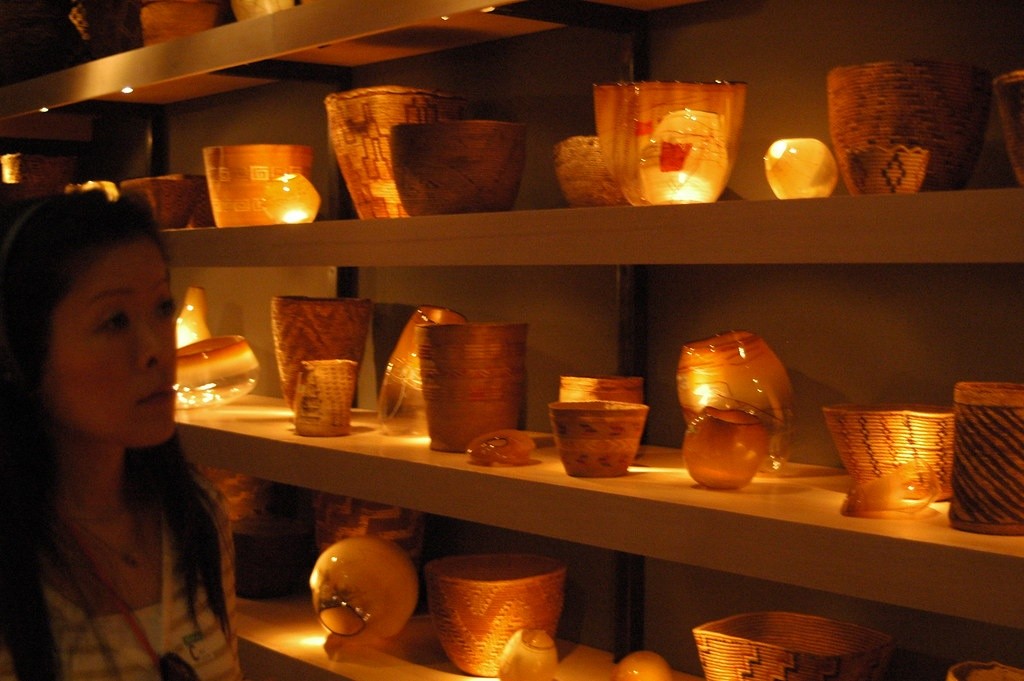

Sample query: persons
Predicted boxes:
[0,195,243,681]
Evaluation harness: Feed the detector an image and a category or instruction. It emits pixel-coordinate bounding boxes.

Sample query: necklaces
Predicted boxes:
[72,518,143,565]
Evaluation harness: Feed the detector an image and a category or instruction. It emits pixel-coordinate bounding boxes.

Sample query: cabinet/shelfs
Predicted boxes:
[0,0,1024,680]
